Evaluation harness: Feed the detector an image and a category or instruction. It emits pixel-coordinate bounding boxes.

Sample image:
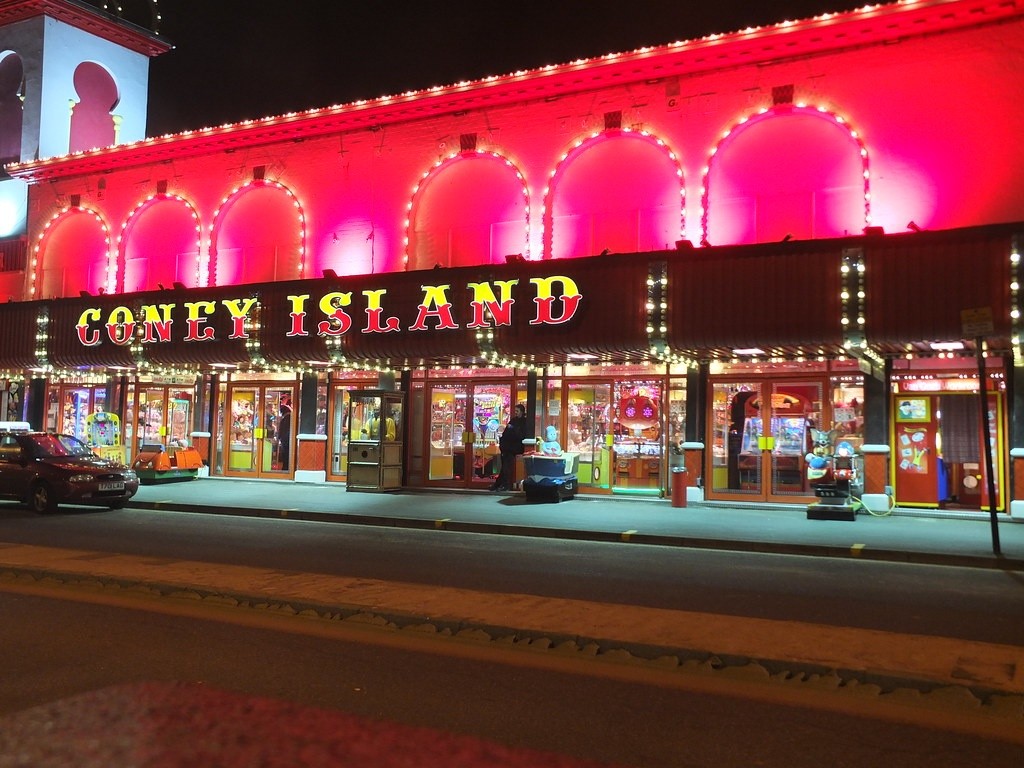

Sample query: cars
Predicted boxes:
[0,420,138,514]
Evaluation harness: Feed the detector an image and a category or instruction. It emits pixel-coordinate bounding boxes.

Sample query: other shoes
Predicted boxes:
[487,484,503,491]
[497,484,513,491]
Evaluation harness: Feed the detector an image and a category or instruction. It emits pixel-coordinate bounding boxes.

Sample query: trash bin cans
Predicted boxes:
[672,466,687,507]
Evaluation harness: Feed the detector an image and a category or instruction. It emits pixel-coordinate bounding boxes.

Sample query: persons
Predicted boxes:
[485,403,528,492]
[279,399,292,471]
[359,399,395,442]
[540,425,563,458]
